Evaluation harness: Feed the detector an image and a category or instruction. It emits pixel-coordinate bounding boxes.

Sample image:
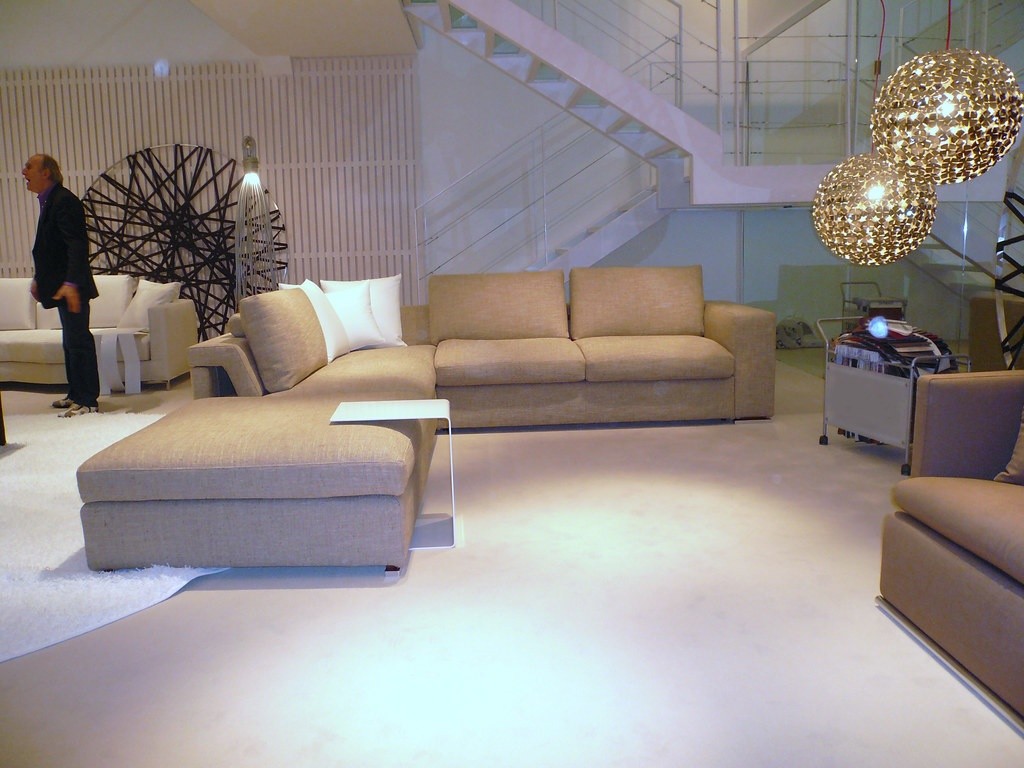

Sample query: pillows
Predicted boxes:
[300,279,350,364]
[327,278,386,349]
[119,279,183,332]
[240,287,328,396]
[322,273,406,347]
[994,411,1024,485]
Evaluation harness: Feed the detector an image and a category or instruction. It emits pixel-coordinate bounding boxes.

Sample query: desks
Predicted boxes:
[330,399,456,553]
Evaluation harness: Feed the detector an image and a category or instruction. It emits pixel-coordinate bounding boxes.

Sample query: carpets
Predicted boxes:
[0,413,229,664]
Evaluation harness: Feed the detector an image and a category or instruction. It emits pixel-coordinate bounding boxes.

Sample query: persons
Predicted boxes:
[22,154,100,417]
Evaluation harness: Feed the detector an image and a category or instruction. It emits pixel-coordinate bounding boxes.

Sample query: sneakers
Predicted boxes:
[58,402,99,417]
[52,395,75,408]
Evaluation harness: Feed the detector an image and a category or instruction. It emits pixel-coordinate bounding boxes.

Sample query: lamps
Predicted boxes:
[812,1,939,267]
[871,0,1024,183]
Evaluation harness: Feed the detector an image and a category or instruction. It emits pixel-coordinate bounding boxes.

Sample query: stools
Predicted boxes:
[74,397,437,580]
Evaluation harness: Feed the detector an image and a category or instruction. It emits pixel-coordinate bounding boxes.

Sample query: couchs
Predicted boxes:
[875,370,1024,768]
[0,276,197,391]
[188,266,776,451]
